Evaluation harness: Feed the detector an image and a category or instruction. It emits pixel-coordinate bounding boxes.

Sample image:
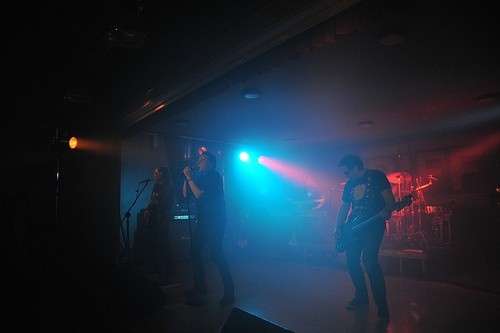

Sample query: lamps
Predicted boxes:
[106,0,148,45]
[64,68,95,106]
[241,81,261,100]
[377,27,407,46]
[46,126,80,155]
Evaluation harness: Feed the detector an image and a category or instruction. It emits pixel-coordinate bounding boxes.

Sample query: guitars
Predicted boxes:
[336,194,413,252]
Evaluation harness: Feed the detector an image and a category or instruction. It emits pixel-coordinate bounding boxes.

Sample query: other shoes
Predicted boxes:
[184,287,208,296]
[376,303,389,322]
[345,287,369,309]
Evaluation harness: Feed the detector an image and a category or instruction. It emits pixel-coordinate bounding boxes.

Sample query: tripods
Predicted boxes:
[412,178,433,248]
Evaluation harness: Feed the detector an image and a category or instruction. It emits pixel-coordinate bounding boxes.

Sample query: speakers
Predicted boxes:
[218,306,295,333]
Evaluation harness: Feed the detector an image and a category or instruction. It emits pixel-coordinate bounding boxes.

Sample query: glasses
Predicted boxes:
[198,157,207,163]
[344,170,349,176]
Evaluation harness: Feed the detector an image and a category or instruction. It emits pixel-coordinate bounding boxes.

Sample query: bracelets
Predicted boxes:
[187,178,194,181]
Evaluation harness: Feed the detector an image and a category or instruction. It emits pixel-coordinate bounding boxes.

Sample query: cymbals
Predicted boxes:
[386,172,411,184]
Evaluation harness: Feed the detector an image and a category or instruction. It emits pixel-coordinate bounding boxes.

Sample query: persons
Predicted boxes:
[333,153,397,322]
[143,167,177,280]
[182,151,238,306]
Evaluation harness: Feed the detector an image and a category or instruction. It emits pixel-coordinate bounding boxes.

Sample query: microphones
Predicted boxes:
[142,178,153,183]
[190,166,197,171]
